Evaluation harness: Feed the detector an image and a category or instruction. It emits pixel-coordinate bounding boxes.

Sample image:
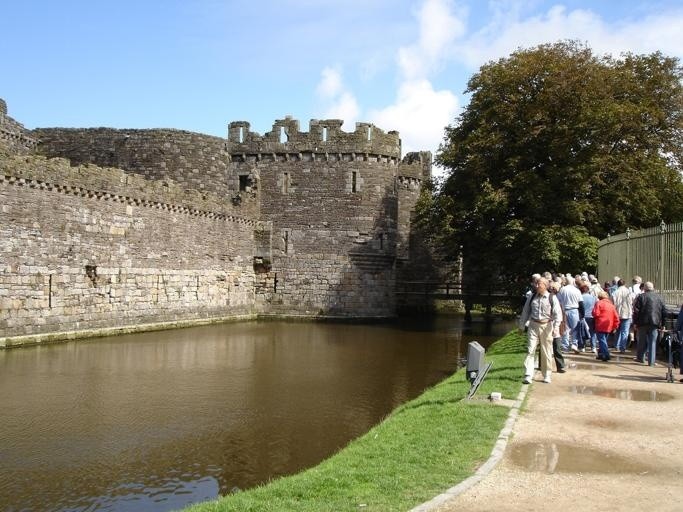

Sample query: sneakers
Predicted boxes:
[557,367,566,373]
[544,376,551,384]
[591,345,611,361]
[522,375,533,384]
[615,346,655,366]
[571,344,585,354]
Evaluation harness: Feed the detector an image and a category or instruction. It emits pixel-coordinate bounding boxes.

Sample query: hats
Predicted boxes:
[581,271,598,283]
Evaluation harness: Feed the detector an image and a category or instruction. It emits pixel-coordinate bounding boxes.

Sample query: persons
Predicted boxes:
[518,270,683,384]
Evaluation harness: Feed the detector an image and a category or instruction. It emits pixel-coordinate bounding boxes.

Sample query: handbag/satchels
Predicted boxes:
[661,331,682,352]
